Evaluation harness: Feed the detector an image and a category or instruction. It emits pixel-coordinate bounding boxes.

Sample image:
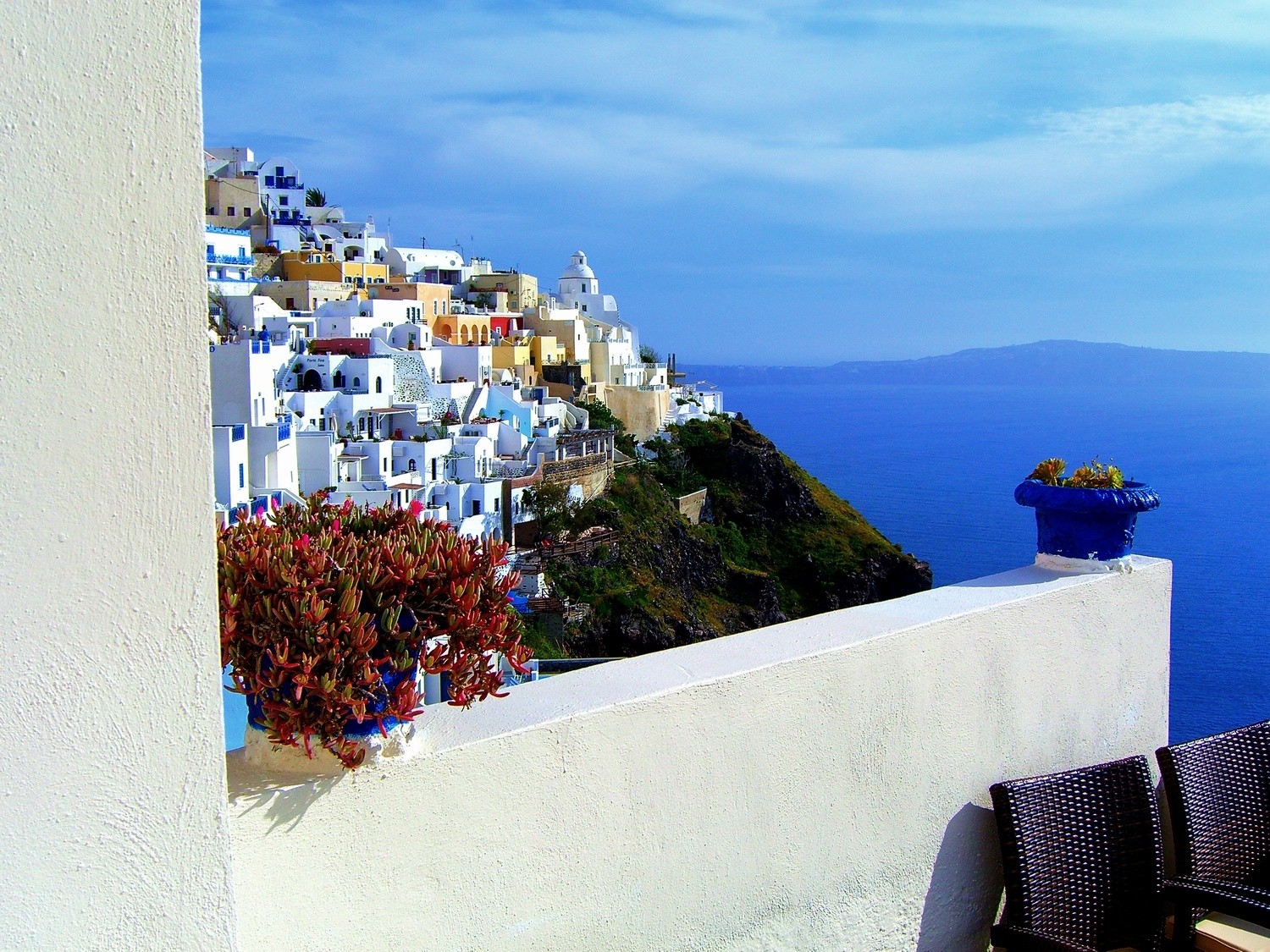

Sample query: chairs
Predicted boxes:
[362,484,377,491]
[988,721,1270,952]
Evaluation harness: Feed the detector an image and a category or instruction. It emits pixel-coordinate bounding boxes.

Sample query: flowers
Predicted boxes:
[217,489,533,773]
[1025,454,1123,490]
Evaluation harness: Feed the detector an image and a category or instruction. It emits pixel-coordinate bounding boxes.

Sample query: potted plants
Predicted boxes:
[407,340,415,351]
[480,339,487,346]
[344,493,353,499]
[324,486,338,493]
[468,341,474,346]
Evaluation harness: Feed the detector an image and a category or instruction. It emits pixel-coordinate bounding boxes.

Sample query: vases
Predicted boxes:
[1014,477,1161,562]
[241,609,423,737]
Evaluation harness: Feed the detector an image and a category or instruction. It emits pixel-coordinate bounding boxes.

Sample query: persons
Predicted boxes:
[257,325,270,341]
